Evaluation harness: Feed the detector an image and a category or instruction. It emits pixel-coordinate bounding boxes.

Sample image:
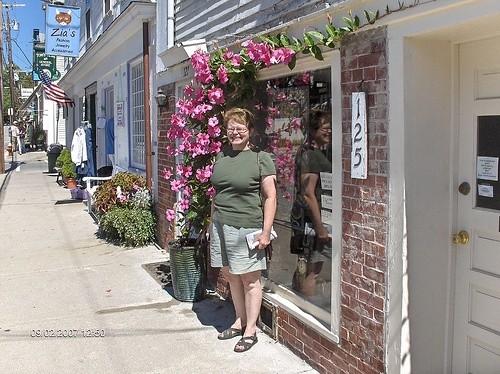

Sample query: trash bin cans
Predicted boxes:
[46,147,61,173]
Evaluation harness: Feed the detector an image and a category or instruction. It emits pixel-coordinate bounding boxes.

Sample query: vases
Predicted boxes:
[169,239,209,302]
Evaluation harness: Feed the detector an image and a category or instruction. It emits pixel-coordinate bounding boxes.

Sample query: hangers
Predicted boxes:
[79,121,91,130]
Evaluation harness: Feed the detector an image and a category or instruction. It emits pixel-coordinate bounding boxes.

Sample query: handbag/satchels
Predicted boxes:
[289,234,324,255]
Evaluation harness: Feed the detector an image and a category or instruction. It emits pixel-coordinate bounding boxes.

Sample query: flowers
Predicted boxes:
[163,0,434,245]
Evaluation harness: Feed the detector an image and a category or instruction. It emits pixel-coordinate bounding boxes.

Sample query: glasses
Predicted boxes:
[320,125,332,132]
[225,128,249,134]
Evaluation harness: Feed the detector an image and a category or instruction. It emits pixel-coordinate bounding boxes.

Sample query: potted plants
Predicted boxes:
[93,170,151,217]
[56,148,77,190]
[95,205,157,248]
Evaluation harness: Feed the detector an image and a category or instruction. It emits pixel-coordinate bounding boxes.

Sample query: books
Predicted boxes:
[304,222,332,237]
[245,226,278,250]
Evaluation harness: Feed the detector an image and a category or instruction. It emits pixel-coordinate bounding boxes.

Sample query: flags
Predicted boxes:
[39,70,75,108]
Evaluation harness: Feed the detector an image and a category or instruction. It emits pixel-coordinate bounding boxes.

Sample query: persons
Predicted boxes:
[207,107,277,352]
[105,118,115,168]
[289,110,332,300]
[11,121,38,155]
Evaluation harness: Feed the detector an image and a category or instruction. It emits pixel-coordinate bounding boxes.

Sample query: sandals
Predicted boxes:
[234,331,259,353]
[217,326,246,340]
